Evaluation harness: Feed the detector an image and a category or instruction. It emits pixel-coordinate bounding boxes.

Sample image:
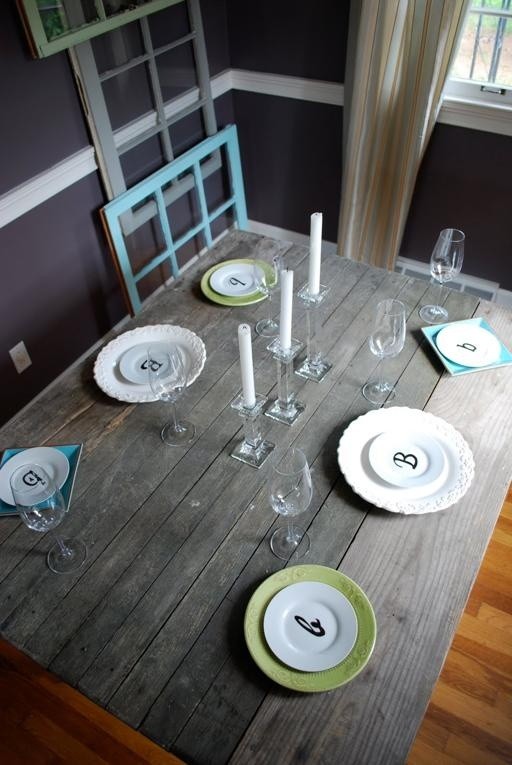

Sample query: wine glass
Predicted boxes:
[146,342,198,447]
[252,238,285,340]
[418,228,465,325]
[360,300,407,404]
[265,448,312,562]
[10,463,87,575]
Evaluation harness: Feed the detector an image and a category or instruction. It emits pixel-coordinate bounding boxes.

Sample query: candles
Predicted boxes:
[309,212,323,295]
[238,324,256,407]
[279,267,293,349]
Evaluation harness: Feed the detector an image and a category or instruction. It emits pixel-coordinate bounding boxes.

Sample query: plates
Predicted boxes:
[0,443,85,514]
[422,317,511,377]
[263,581,360,673]
[93,324,206,405]
[201,258,278,305]
[436,324,500,368]
[244,563,377,695]
[337,405,475,517]
[368,429,444,486]
[119,342,180,385]
[209,263,264,297]
[0,447,70,506]
